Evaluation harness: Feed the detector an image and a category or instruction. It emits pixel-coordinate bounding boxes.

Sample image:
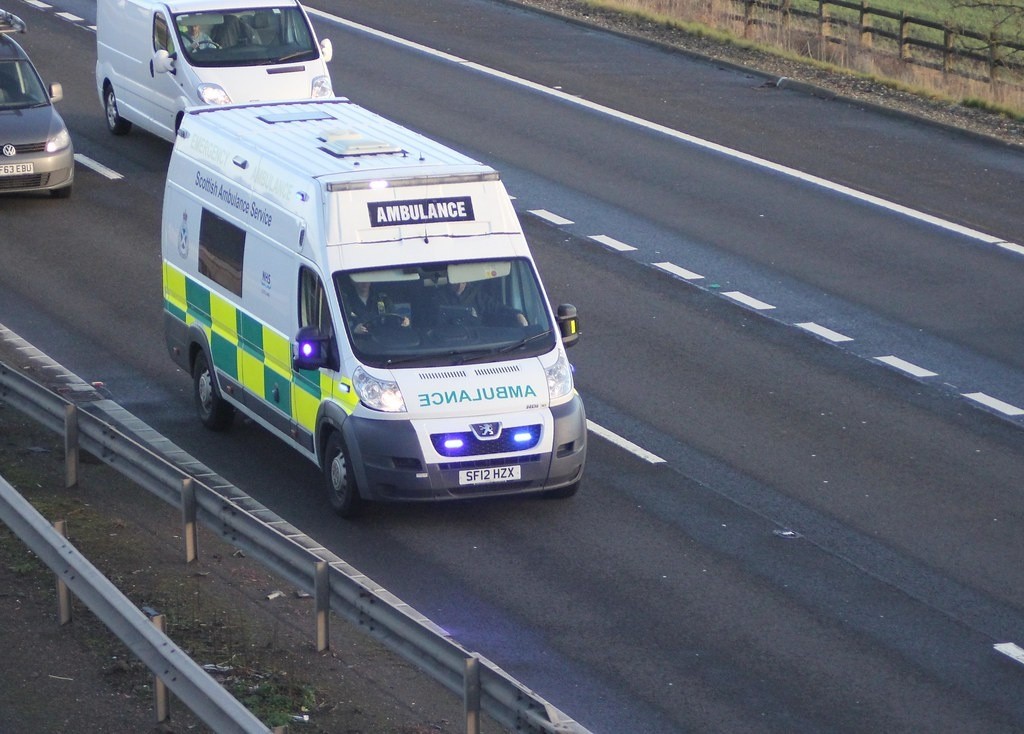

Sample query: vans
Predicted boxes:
[95,1,335,143]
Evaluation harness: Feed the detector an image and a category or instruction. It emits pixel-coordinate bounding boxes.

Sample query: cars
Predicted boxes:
[1,33,74,198]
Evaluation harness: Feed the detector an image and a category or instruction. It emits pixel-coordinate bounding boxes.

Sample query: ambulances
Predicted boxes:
[161,94,587,518]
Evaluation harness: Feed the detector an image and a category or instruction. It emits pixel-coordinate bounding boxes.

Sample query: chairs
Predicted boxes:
[250,13,279,46]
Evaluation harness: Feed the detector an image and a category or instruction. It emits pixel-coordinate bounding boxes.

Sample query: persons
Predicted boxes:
[431,282,529,329]
[181,25,218,54]
[343,281,410,334]
